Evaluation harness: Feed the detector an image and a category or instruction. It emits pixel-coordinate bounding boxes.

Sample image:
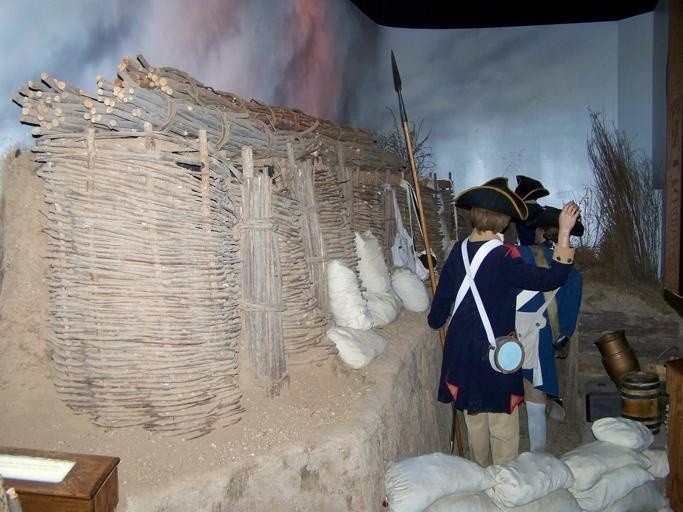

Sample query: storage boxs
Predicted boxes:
[0,445,122,512]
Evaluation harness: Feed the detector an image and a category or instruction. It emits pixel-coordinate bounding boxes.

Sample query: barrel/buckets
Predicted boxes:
[594,329,629,355]
[602,353,640,382]
[619,370,663,433]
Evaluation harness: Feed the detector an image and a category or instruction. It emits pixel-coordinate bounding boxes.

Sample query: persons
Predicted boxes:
[427,175,584,468]
